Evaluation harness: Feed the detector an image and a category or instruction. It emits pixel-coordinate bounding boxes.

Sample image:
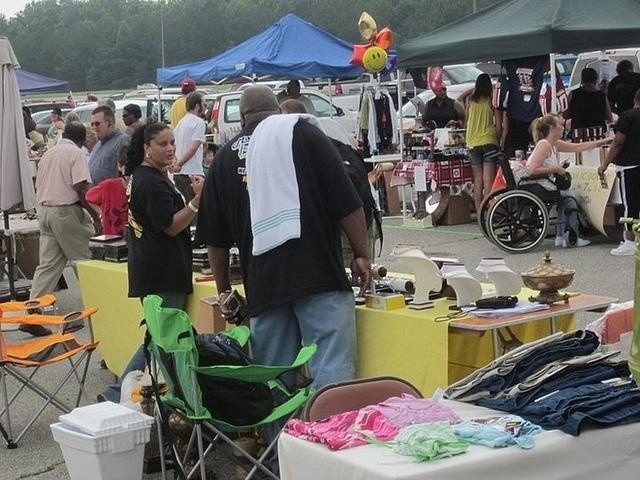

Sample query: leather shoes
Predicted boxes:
[18,324,51,335]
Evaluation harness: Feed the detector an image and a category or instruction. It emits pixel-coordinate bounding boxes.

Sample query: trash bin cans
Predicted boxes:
[50,401,155,480]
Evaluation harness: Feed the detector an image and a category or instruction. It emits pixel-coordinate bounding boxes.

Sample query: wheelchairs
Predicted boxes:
[478,149,578,253]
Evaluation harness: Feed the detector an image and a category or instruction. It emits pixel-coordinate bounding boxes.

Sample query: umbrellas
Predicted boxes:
[395,0,639,68]
[16,67,72,95]
[0,37,38,300]
[156,15,396,82]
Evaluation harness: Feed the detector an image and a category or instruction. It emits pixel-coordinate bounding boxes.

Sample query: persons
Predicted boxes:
[86,146,132,235]
[563,68,612,140]
[608,59,640,115]
[98,99,126,132]
[279,81,317,116]
[47,107,65,142]
[88,106,129,185]
[167,92,209,250]
[19,120,96,336]
[521,114,614,248]
[170,79,197,129]
[281,100,379,267]
[422,84,468,131]
[122,105,145,139]
[98,122,204,405]
[197,85,373,478]
[497,56,542,158]
[598,89,640,257]
[464,73,502,222]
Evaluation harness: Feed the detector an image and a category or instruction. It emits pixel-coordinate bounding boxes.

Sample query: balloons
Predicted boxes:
[348,11,395,75]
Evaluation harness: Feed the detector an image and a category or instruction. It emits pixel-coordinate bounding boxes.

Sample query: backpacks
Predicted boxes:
[194,334,272,425]
[329,137,383,259]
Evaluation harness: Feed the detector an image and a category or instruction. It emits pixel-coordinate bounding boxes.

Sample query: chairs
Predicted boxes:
[0,293,100,449]
[139,294,317,480]
[304,375,424,423]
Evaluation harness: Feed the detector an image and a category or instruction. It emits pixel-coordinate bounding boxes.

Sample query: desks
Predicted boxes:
[396,160,473,223]
[278,334,640,480]
[73,260,619,384]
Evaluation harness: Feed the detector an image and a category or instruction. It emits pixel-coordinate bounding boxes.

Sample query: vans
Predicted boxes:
[236,80,308,93]
[394,83,480,128]
[435,63,485,84]
[211,89,359,158]
[62,99,159,129]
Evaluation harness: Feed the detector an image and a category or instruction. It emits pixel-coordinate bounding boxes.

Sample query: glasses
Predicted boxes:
[91,121,105,126]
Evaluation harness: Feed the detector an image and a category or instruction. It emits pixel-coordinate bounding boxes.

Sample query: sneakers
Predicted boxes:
[610,241,637,256]
[555,238,563,246]
[563,237,590,249]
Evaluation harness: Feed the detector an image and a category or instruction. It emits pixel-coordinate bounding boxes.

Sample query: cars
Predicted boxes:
[33,110,71,134]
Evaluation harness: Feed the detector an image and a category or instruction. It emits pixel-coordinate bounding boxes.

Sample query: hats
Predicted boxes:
[180,78,195,90]
